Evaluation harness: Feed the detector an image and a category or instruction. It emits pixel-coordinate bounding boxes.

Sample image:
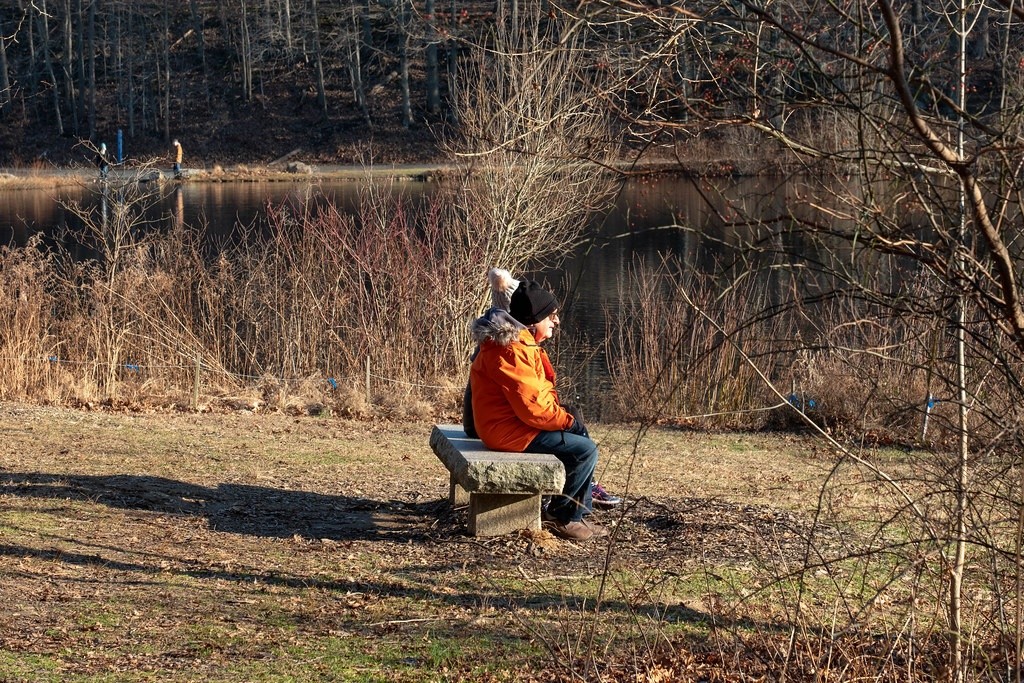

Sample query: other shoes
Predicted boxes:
[579,518,612,537]
[541,511,593,540]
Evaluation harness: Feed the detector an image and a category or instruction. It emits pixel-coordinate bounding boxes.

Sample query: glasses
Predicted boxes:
[549,312,560,320]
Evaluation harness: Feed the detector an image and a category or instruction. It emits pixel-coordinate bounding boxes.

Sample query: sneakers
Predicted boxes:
[590,480,620,504]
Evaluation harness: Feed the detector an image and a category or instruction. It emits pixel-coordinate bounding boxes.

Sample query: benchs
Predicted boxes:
[429,425,565,536]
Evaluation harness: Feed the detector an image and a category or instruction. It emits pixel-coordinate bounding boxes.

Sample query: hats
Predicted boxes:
[488,267,521,313]
[508,279,561,326]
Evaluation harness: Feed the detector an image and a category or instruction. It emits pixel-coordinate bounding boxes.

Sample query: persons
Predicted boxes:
[471,282,611,541]
[173,139,182,177]
[97,144,110,181]
[464,267,621,520]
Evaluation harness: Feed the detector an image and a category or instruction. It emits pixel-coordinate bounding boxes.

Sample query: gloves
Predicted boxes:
[559,403,585,431]
[565,420,590,439]
